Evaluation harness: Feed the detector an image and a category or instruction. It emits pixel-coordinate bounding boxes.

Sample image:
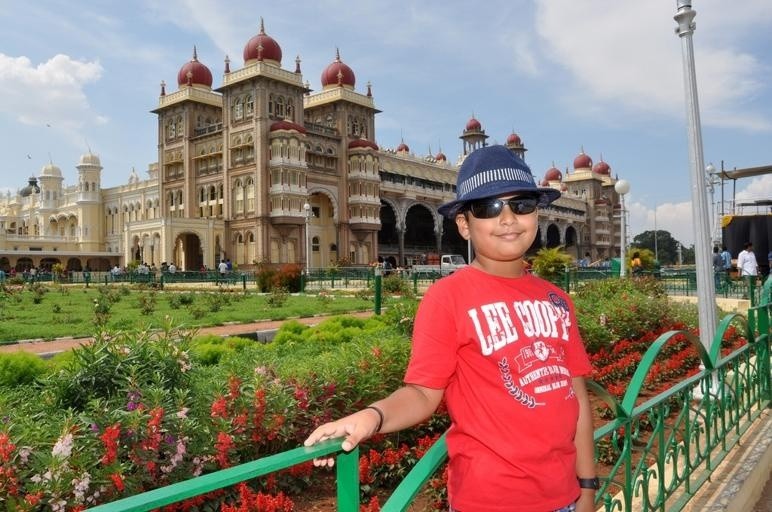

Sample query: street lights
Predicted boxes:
[303,203,311,276]
[615,180,629,277]
[705,162,718,253]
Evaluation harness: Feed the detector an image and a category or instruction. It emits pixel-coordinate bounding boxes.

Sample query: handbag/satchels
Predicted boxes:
[756,280,762,286]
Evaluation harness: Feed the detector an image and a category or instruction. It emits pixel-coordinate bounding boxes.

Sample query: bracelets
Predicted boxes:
[575,475,600,491]
[364,406,384,434]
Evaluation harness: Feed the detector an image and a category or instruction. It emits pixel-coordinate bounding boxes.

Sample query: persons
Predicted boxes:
[736,242,762,290]
[8,265,48,281]
[652,255,662,280]
[304,144,601,512]
[631,251,644,278]
[720,246,738,291]
[199,257,233,278]
[110,262,176,279]
[712,246,725,290]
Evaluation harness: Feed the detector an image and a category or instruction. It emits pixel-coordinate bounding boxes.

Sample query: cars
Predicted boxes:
[728,259,763,287]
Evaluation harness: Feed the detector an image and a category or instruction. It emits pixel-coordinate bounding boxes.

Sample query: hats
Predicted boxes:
[438,145,561,219]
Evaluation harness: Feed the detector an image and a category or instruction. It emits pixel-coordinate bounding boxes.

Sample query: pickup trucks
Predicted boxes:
[412,255,468,278]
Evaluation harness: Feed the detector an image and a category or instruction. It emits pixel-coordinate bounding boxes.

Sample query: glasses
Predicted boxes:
[460,193,536,218]
